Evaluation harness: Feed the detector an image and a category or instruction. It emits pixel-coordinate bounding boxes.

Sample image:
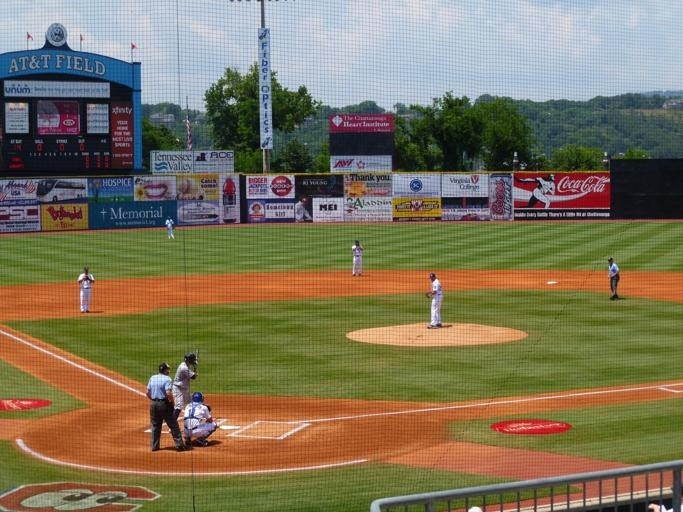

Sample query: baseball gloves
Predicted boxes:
[202,404,211,414]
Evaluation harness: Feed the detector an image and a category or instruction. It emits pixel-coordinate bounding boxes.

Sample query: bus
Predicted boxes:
[35,180,87,203]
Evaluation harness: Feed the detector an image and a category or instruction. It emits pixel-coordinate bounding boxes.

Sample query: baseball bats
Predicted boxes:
[195,348,199,367]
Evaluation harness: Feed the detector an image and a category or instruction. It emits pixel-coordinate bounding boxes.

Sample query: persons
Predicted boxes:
[77,266,94,313]
[164,215,174,240]
[294,198,312,223]
[144,351,218,451]
[352,239,364,277]
[250,203,263,216]
[608,257,620,299]
[517,173,556,209]
[425,273,443,329]
[142,180,173,199]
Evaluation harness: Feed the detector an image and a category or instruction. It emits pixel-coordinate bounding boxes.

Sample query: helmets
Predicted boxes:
[159,352,202,402]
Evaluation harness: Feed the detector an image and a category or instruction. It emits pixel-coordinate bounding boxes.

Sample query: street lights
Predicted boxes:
[513,152,520,171]
[604,152,610,171]
